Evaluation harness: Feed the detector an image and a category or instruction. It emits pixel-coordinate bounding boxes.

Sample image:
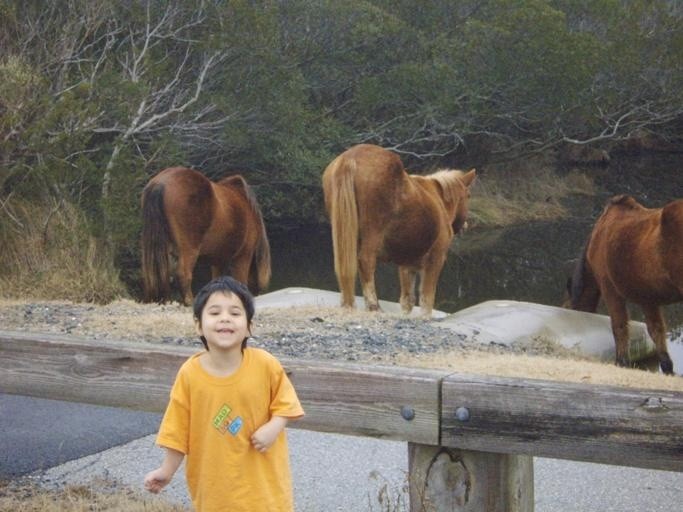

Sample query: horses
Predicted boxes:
[140,166,271,307]
[571,195,683,375]
[322,144,476,320]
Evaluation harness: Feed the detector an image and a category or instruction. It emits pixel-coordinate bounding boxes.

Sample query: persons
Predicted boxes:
[142,274,305,512]
